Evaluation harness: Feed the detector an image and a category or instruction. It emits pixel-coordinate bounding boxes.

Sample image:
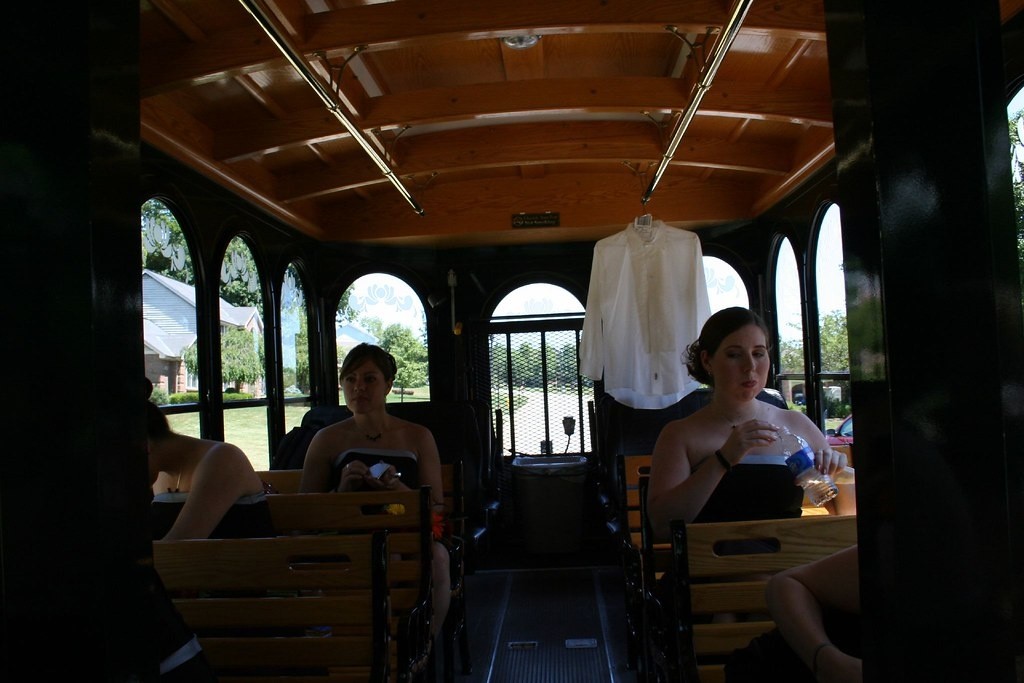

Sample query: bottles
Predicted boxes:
[777,425,840,507]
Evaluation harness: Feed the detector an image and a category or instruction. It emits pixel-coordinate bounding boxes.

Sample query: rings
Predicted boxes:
[347,463,354,471]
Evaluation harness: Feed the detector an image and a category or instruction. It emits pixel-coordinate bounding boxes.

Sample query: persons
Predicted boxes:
[643,306,849,660]
[724,545,863,683]
[142,376,331,679]
[300,344,452,672]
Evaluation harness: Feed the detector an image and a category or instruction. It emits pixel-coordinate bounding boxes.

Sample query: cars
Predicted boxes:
[824,413,853,446]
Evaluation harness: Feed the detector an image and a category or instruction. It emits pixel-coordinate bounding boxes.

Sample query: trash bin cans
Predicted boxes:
[510,455,589,558]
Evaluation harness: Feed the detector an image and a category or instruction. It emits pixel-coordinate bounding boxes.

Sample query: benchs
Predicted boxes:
[621,446,859,683]
[150,458,470,683]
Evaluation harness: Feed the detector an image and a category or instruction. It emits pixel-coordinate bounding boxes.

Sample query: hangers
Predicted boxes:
[637,198,657,234]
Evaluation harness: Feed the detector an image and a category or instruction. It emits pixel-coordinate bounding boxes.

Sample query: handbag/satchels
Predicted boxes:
[723,625,818,683]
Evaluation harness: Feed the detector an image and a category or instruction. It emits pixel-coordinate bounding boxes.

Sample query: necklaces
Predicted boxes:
[162,469,184,493]
[353,418,388,440]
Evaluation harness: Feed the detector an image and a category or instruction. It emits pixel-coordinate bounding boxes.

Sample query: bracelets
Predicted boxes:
[715,449,732,472]
[813,641,837,677]
